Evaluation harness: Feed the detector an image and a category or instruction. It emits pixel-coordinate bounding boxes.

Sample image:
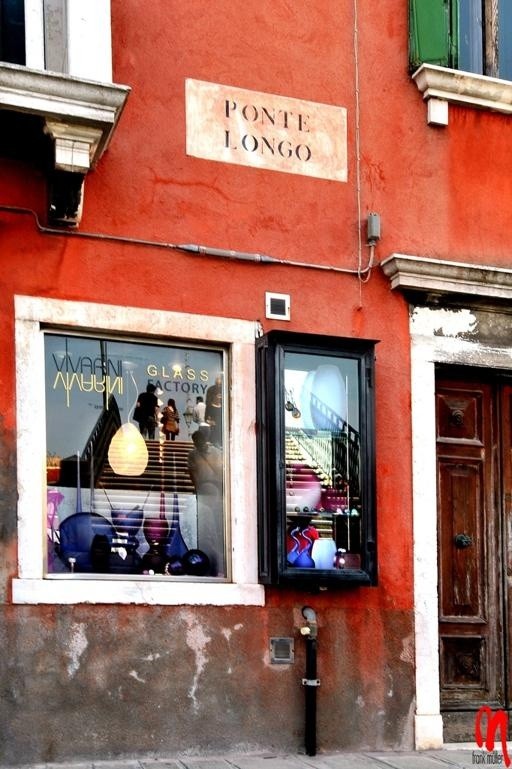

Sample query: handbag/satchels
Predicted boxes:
[133,407,141,423]
[161,417,167,423]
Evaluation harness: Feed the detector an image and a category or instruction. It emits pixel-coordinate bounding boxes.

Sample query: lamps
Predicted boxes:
[107,361,149,476]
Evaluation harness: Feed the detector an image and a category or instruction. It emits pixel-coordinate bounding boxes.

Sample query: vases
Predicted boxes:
[164,494,189,563]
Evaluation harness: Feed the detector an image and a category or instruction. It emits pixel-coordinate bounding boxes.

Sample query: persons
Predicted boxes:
[184,399,194,429]
[136,383,161,439]
[206,376,222,405]
[159,397,181,441]
[192,396,212,443]
[204,392,223,450]
[186,432,223,498]
